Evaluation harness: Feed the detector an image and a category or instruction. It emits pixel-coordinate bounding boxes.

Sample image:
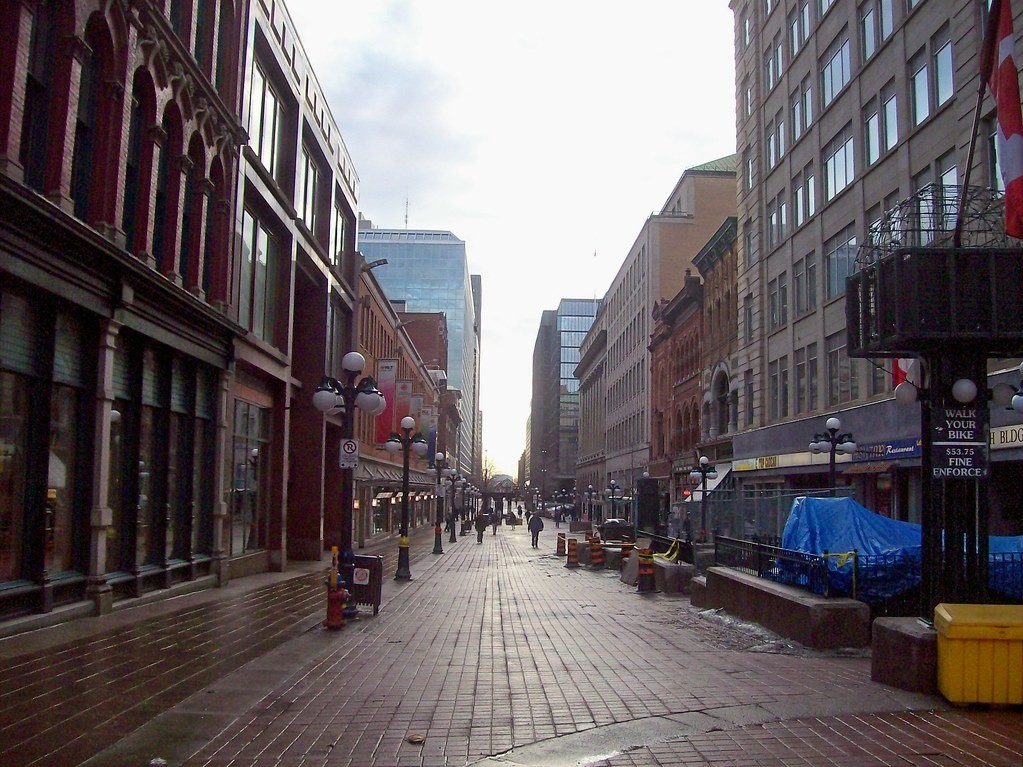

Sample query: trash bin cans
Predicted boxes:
[464,520,472,530]
[338,553,386,616]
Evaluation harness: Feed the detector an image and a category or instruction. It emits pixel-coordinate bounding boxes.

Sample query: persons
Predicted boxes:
[525,510,530,524]
[555,510,560,528]
[510,512,515,530]
[444,512,450,532]
[491,512,499,535]
[528,512,543,548]
[475,515,486,544]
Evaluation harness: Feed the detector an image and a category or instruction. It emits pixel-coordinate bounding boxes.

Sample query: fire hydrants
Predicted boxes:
[323,572,351,629]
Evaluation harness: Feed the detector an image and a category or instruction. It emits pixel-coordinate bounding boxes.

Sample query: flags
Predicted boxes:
[980,0,1023,240]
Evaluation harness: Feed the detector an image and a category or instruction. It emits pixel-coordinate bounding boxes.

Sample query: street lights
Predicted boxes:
[570,487,579,516]
[606,480,620,520]
[584,485,596,521]
[314,351,386,622]
[551,490,560,513]
[690,455,718,543]
[385,416,428,581]
[427,451,447,555]
[559,488,569,522]
[444,469,462,543]
[809,417,857,497]
[457,478,481,536]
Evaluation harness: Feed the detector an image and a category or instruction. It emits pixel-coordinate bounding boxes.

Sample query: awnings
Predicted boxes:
[684,463,732,505]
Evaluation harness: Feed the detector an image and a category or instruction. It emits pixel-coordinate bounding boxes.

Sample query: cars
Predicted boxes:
[598,518,633,539]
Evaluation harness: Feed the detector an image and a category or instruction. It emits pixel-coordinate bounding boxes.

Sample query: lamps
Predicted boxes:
[989,384,1019,405]
[394,346,403,356]
[360,294,370,308]
[894,383,928,404]
[952,380,977,403]
